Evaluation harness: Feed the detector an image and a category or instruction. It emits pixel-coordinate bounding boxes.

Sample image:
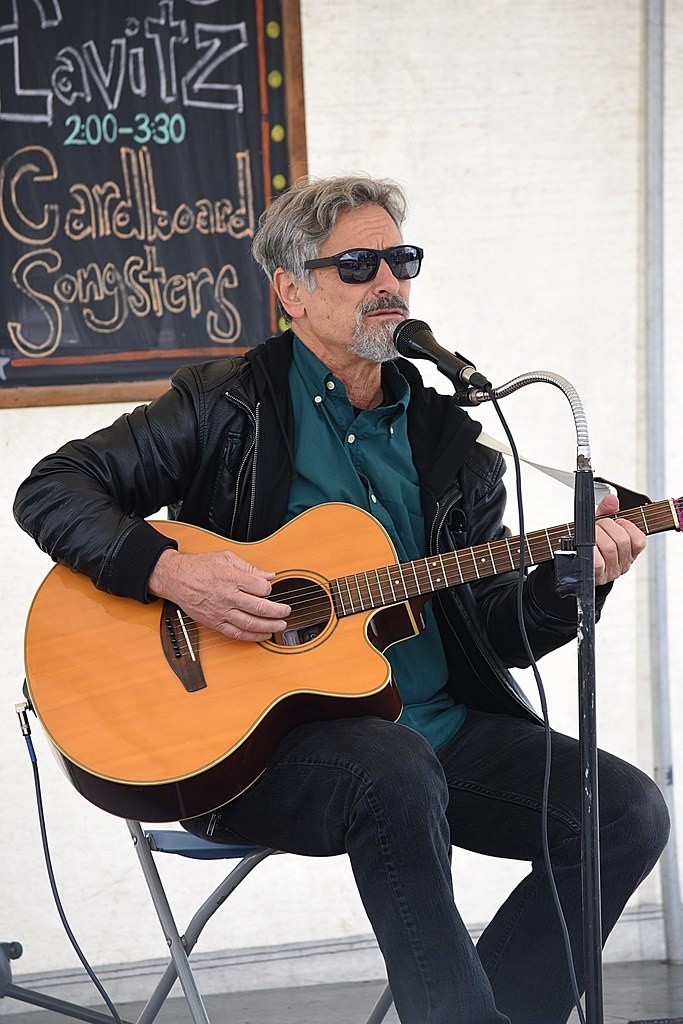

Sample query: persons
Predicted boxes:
[10,177,672,1023]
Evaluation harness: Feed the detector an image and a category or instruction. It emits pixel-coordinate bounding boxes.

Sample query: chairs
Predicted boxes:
[126,819,394,1024]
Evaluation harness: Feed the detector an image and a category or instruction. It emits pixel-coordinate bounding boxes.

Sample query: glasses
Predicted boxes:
[298,245,424,285]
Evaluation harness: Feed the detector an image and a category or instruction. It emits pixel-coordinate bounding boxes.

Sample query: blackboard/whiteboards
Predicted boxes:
[0,0,310,410]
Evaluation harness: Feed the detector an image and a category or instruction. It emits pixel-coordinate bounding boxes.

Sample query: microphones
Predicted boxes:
[393,318,494,391]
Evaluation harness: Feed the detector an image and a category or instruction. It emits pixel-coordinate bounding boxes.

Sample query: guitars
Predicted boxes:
[22,494,682,825]
